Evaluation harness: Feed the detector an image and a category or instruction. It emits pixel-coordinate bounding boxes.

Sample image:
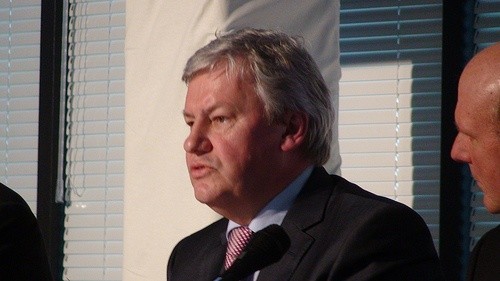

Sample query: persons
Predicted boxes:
[167,26,444,281]
[0,183,53,281]
[449,44,500,281]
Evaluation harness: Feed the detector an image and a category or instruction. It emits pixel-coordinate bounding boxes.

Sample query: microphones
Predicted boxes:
[213,224,291,281]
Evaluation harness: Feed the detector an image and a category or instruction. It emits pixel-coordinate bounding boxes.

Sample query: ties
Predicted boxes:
[224,225,253,272]
